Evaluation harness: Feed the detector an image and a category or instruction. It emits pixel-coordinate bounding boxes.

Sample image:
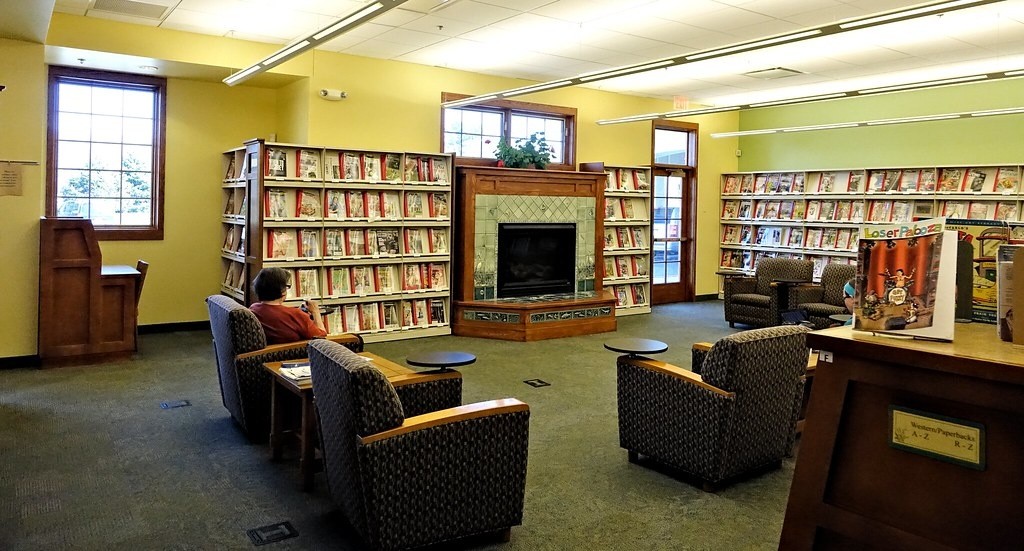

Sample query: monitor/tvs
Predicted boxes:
[498,225,575,297]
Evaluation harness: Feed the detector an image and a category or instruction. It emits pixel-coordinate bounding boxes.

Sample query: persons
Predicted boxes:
[249,267,327,345]
[842,278,855,326]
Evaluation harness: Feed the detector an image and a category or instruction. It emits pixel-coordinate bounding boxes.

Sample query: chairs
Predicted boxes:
[306,339,530,551]
[204,294,364,444]
[134,260,148,352]
[716,258,814,327]
[773,264,857,328]
[604,325,814,493]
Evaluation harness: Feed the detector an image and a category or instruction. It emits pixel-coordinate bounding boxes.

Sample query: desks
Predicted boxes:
[776,321,1024,551]
[36,216,142,369]
[222,138,452,344]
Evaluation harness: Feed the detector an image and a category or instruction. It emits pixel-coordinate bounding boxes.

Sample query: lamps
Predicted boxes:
[438,0,1003,109]
[595,69,1024,125]
[222,0,410,87]
[320,89,349,101]
[710,106,1024,140]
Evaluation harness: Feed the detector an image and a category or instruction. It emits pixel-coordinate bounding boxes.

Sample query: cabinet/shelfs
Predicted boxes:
[603,166,651,317]
[718,164,1024,299]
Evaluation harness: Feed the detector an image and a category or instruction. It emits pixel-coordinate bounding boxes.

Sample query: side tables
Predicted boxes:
[262,352,418,493]
[795,348,819,433]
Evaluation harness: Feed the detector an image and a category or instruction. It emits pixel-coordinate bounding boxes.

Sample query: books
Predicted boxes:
[937,167,1024,244]
[603,169,650,309]
[325,153,399,335]
[867,168,935,223]
[222,149,246,305]
[266,147,324,326]
[720,174,753,292]
[852,216,1024,345]
[279,361,313,386]
[752,173,804,269]
[804,172,864,278]
[402,155,449,327]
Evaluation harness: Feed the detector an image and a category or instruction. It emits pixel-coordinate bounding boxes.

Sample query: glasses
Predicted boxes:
[285,283,292,289]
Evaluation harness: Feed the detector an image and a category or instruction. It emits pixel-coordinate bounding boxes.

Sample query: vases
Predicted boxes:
[527,161,539,169]
[497,160,507,167]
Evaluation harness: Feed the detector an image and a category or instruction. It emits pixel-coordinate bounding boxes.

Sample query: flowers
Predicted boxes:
[486,132,558,170]
